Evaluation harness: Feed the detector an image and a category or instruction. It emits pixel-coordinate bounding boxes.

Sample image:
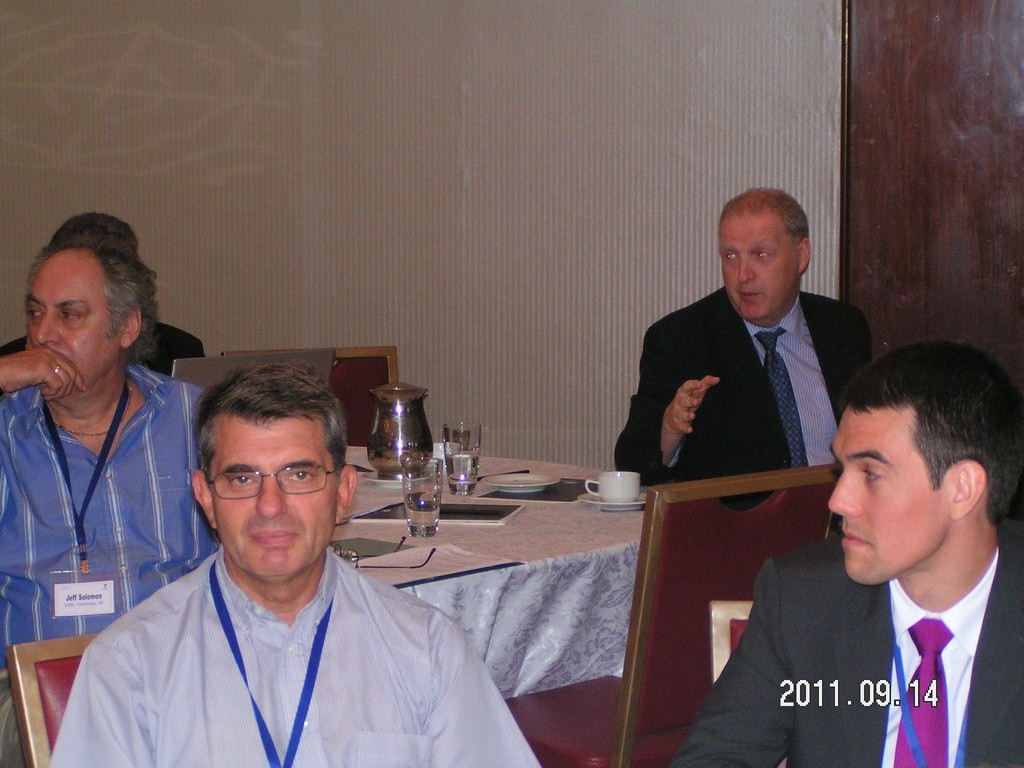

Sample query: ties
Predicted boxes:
[755,327,807,468]
[893,617,955,768]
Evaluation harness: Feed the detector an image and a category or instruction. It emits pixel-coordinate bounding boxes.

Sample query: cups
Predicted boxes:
[441,422,482,495]
[585,471,640,502]
[427,443,460,475]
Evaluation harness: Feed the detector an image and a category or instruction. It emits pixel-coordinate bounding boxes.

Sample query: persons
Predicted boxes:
[667,340,1024,768]
[0,212,208,376]
[614,186,872,510]
[50,355,540,768]
[0,229,217,767]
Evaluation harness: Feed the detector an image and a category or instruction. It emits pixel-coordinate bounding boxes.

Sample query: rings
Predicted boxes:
[55,365,60,373]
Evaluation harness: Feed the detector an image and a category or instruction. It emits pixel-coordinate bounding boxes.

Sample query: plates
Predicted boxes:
[577,493,647,510]
[480,473,560,492]
[361,472,427,488]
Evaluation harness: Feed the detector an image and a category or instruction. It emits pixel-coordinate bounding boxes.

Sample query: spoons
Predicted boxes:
[448,470,530,481]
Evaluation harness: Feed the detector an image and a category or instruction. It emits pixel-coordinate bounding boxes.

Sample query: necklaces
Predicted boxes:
[56,383,133,436]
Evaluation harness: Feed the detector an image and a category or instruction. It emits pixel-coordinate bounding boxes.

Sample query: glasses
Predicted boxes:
[334,536,436,569]
[207,465,345,500]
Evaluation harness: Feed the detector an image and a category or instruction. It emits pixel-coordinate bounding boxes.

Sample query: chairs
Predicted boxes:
[6,634,99,768]
[507,466,834,768]
[224,344,397,447]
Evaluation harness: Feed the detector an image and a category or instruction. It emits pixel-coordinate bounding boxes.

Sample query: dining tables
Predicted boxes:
[333,445,645,705]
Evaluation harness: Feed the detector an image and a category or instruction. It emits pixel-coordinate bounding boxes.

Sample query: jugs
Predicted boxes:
[369,382,433,481]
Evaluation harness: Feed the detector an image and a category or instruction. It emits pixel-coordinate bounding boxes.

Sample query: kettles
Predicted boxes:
[401,457,443,538]
